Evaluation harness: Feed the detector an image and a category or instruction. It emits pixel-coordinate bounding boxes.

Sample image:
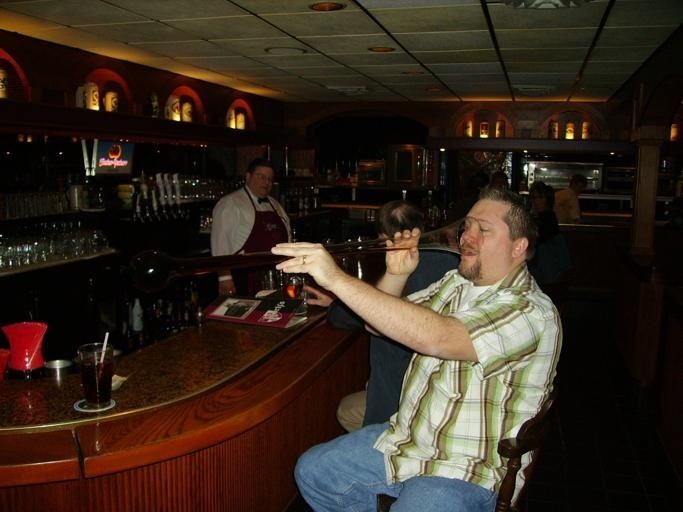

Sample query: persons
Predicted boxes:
[553,175,588,224]
[210,160,291,296]
[271,171,563,512]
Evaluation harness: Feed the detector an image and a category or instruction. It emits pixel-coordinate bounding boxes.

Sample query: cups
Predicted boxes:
[280,273,309,318]
[0,183,103,269]
[77,343,113,408]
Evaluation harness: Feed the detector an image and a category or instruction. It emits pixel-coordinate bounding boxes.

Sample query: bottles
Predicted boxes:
[133,180,204,350]
[286,185,320,213]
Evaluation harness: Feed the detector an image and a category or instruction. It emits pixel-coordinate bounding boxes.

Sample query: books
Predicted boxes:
[203,295,308,329]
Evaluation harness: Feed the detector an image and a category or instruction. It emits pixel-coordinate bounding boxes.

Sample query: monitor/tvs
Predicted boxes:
[80,138,91,177]
[92,139,134,176]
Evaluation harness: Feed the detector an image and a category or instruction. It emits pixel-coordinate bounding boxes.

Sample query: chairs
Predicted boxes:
[376,391,556,512]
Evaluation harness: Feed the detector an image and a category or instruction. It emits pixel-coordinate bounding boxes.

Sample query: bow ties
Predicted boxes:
[257,196,270,204]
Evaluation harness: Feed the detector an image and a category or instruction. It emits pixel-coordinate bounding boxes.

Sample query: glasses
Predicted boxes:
[251,171,274,182]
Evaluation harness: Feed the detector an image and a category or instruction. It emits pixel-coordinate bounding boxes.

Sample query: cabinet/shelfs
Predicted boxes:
[0,95,673,279]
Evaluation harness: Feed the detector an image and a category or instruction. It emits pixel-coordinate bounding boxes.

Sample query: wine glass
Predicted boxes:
[132,216,483,295]
